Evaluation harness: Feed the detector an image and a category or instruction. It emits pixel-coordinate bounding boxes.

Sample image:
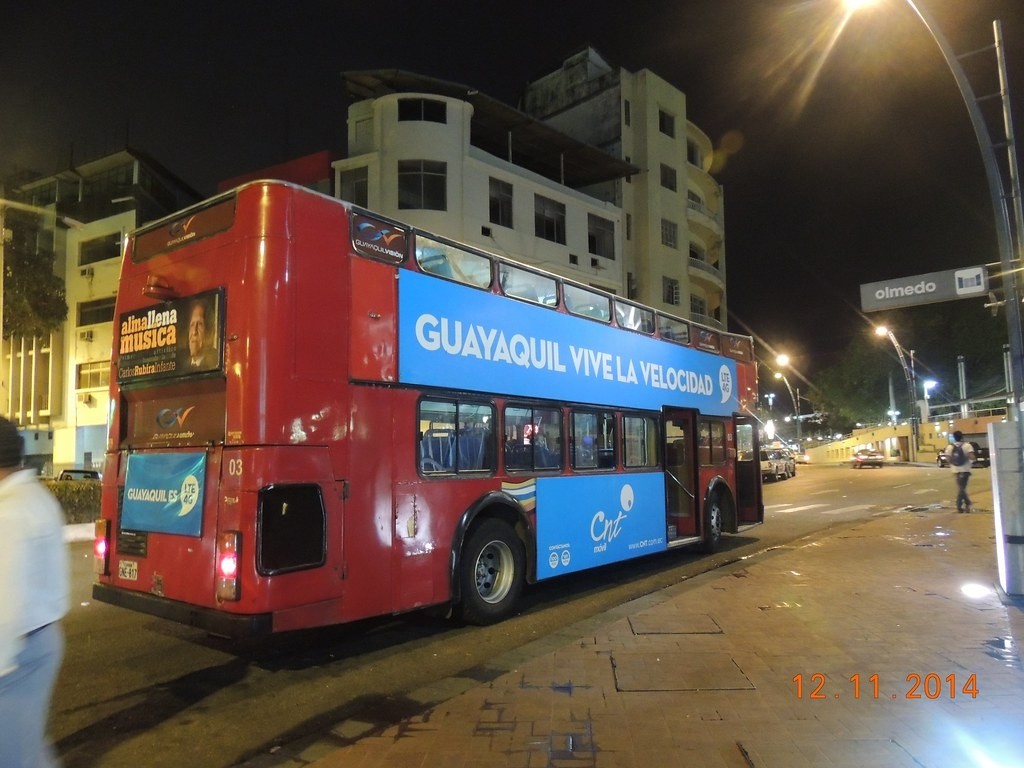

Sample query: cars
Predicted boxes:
[737,443,796,482]
[935,441,981,467]
[851,448,884,469]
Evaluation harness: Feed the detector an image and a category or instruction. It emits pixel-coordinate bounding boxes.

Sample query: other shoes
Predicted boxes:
[957,506,963,512]
[966,501,972,512]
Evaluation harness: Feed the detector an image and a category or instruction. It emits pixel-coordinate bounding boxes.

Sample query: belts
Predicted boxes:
[18,622,55,639]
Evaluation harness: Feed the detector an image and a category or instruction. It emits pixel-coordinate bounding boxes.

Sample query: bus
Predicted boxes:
[90,174,767,643]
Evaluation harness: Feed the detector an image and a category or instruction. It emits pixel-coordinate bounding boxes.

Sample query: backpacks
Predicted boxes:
[951,442,967,466]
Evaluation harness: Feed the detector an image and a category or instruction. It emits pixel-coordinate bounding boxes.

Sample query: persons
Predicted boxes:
[182,299,218,372]
[527,432,560,467]
[945,430,976,513]
[0,416,71,768]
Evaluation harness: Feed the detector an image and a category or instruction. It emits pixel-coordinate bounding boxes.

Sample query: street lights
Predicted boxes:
[877,325,920,452]
[910,349,917,418]
[923,381,936,417]
[774,372,800,439]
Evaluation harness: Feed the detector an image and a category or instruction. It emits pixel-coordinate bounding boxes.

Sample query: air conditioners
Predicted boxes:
[81,268,94,277]
[81,392,92,403]
[80,331,92,341]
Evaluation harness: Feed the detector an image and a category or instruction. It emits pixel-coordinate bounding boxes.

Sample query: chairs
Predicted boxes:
[65,474,73,480]
[419,428,617,470]
[83,475,90,478]
[416,254,677,342]
